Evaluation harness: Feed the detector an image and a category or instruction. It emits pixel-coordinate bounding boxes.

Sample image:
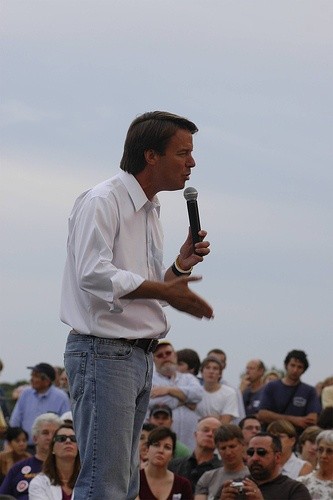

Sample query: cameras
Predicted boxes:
[229,481,246,493]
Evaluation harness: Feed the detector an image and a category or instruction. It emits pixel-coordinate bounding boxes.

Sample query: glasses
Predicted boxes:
[53,434,77,443]
[155,351,173,359]
[246,446,268,457]
[152,414,168,421]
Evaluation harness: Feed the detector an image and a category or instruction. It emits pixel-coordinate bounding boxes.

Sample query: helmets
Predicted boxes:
[60,410,73,422]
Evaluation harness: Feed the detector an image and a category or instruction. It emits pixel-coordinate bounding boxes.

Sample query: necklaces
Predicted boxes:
[156,491,161,496]
[62,479,69,482]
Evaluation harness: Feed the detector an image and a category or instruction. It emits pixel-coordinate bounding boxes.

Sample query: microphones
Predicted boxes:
[184,187,205,256]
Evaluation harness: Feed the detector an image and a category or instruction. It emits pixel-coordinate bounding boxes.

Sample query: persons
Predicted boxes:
[60,111,215,500]
[0,340,333,500]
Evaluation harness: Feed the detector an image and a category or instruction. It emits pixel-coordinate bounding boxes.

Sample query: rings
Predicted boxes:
[254,489,257,493]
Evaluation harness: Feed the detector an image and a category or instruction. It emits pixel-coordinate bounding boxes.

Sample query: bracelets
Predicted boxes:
[171,260,193,277]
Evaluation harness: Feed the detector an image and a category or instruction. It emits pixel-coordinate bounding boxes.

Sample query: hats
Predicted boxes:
[202,356,223,371]
[26,363,56,381]
[148,403,173,417]
[158,339,171,346]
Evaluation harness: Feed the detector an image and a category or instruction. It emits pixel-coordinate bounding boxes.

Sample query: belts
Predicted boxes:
[118,338,160,354]
